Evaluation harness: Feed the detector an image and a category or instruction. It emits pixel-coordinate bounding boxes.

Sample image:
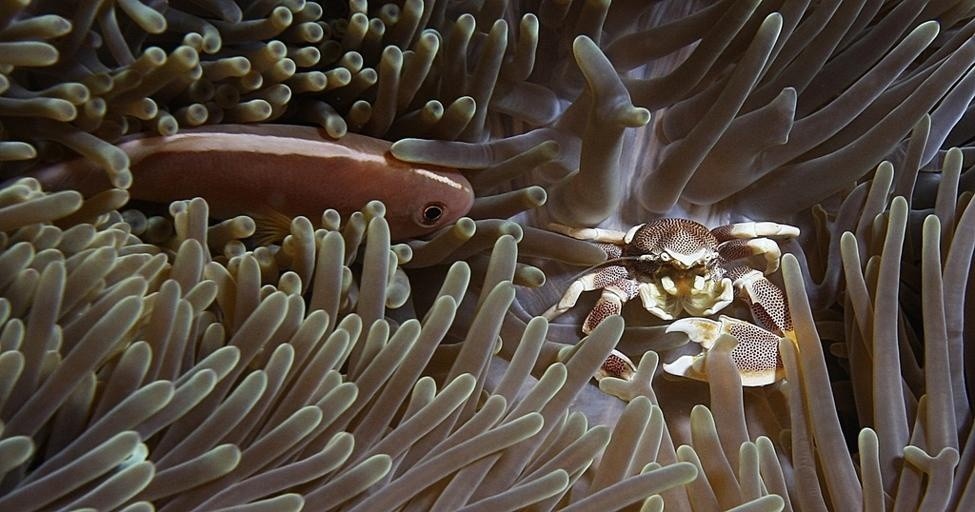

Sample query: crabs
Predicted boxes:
[539,217,800,390]
